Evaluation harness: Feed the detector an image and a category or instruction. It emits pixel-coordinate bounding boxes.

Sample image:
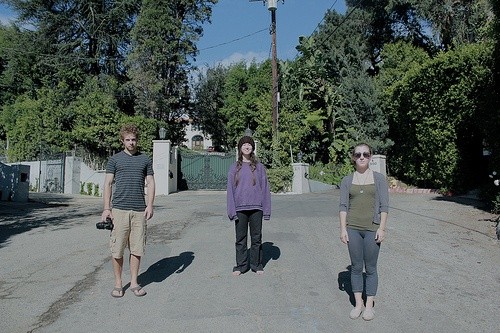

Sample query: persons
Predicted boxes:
[339,143,388,321]
[102,125,155,297]
[227,135,272,277]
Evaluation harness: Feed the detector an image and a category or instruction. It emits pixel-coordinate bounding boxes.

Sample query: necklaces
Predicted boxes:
[356,172,368,194]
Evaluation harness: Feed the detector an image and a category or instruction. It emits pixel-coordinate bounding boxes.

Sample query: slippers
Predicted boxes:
[130,286,146,296]
[111,286,123,297]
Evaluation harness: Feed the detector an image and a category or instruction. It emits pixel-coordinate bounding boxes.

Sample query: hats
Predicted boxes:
[239,136,255,152]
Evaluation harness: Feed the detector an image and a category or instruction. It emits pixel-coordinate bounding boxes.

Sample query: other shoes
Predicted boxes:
[350,301,363,319]
[363,299,376,320]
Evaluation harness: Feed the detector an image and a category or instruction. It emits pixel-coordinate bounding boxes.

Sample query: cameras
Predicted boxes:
[96,216,114,230]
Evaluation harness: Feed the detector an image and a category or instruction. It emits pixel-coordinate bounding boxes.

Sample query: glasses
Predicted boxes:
[353,152,370,159]
